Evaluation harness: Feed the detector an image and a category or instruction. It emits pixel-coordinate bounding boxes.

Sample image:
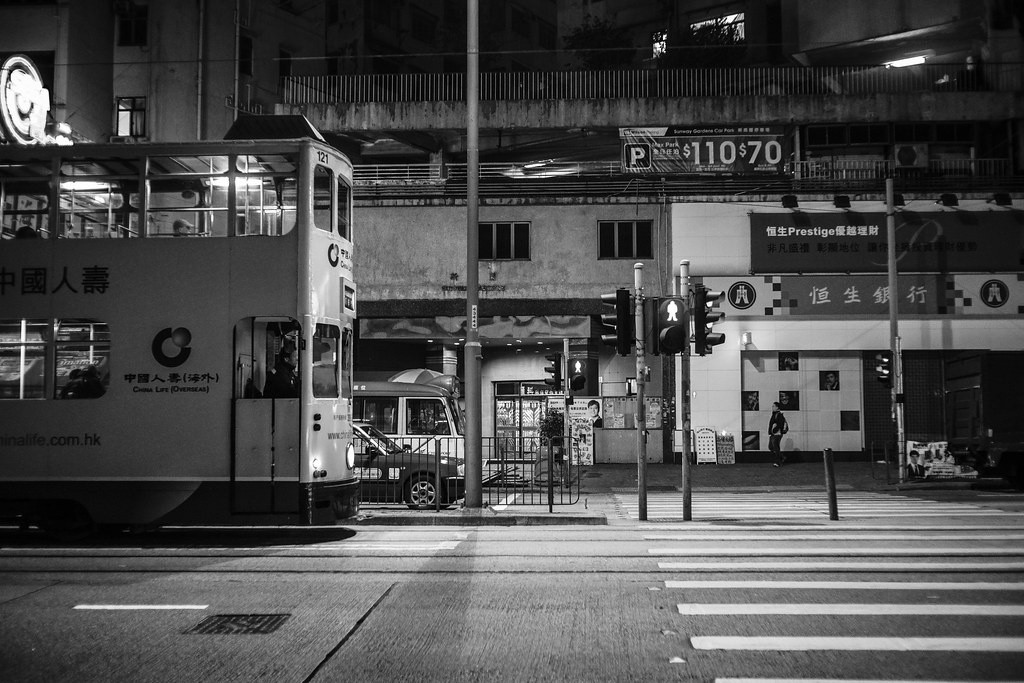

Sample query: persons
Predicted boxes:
[768,402,787,468]
[262,341,298,398]
[780,396,794,410]
[746,391,759,411]
[60,363,106,399]
[421,408,439,435]
[821,371,839,390]
[588,400,602,428]
[15,226,38,240]
[784,356,798,371]
[172,218,193,238]
[907,450,925,478]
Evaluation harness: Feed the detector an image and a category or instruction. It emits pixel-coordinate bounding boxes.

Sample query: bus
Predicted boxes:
[1,113,363,522]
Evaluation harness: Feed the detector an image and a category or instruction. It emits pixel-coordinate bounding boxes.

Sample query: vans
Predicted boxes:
[352,378,466,456]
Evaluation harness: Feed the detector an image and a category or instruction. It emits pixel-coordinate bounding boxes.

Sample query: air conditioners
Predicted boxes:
[893,142,930,167]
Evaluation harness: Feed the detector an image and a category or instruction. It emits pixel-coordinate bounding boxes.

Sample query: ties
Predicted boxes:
[914,465,918,476]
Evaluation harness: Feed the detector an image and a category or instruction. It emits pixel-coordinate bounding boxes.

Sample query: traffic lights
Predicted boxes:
[693,281,726,357]
[567,357,603,400]
[655,295,689,355]
[602,286,633,357]
[544,351,562,391]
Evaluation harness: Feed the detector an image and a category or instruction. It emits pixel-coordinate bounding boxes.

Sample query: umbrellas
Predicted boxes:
[388,367,445,385]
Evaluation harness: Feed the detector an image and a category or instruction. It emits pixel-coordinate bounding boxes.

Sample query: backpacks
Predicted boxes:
[775,412,789,434]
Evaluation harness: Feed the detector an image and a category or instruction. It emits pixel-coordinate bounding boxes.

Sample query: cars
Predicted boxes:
[352,418,466,511]
